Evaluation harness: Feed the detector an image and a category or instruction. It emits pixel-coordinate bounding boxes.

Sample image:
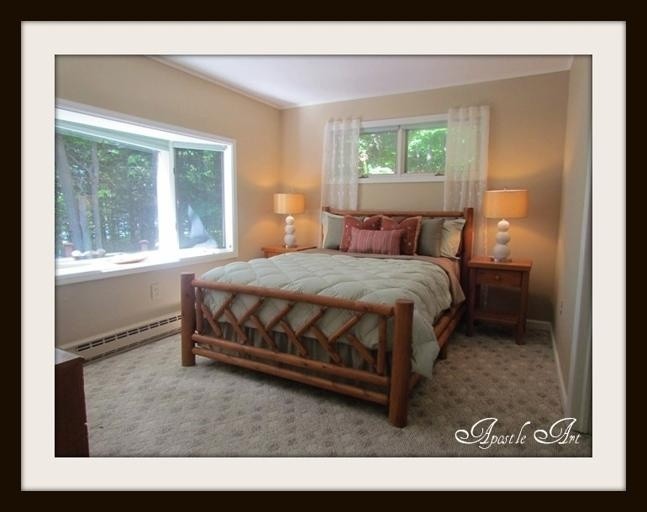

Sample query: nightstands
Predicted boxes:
[260,244,314,259]
[465,255,533,345]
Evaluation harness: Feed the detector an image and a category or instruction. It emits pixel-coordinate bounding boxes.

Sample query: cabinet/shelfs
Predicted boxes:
[55,347,89,457]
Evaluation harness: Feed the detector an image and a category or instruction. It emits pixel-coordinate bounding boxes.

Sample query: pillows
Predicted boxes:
[321,212,467,260]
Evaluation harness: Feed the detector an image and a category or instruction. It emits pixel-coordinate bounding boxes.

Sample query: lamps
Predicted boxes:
[272,193,305,247]
[484,187,529,263]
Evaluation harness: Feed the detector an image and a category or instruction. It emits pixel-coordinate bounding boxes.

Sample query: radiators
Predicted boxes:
[57,312,182,365]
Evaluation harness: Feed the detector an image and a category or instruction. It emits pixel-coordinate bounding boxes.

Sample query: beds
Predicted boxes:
[180,207,475,427]
